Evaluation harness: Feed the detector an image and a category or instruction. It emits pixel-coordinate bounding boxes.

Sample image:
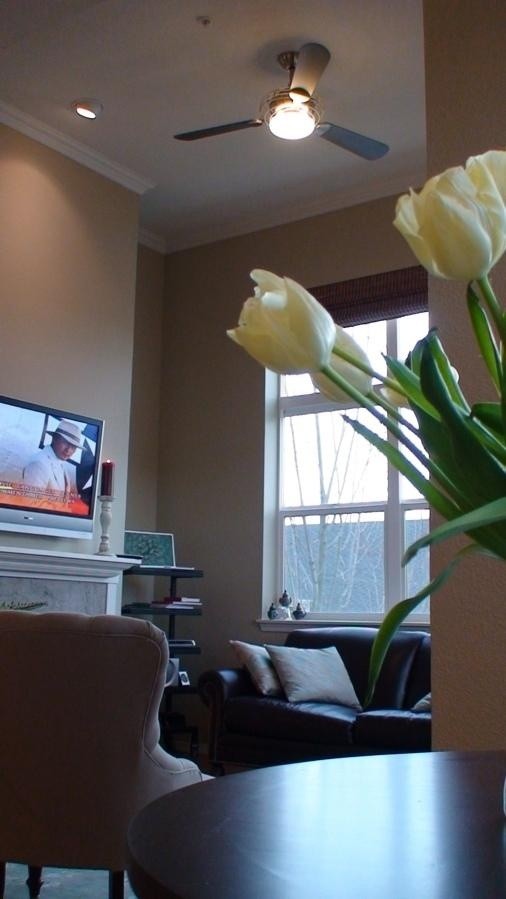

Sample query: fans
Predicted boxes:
[173,43,389,162]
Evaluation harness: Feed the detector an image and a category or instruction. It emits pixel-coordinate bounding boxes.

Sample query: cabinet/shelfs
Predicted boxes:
[123,569,203,758]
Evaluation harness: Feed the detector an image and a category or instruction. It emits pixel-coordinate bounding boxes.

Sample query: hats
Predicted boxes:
[45,420,88,452]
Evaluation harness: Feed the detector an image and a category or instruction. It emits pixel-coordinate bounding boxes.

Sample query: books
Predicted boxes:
[149,595,203,611]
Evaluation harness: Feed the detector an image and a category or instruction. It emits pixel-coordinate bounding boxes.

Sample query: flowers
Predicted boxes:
[227,147,505,706]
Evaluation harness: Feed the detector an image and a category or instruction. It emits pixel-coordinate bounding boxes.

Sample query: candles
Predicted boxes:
[101,459,113,495]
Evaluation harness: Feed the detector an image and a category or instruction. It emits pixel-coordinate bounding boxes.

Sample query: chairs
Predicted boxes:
[1,610,203,898]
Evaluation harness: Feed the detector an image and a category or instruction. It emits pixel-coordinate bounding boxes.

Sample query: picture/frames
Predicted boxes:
[125,530,176,566]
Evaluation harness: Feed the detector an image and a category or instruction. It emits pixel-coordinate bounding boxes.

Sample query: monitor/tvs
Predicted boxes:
[0,393,105,540]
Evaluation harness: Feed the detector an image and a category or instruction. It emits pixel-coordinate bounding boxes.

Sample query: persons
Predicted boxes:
[21,419,88,508]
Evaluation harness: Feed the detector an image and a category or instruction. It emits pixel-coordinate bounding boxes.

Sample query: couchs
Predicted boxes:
[197,627,431,762]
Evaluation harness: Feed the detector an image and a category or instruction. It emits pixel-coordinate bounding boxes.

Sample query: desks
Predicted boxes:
[127,751,506,899]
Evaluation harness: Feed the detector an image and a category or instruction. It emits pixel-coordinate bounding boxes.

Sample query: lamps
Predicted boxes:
[269,107,316,141]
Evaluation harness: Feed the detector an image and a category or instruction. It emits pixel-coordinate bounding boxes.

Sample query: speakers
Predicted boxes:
[165,658,180,688]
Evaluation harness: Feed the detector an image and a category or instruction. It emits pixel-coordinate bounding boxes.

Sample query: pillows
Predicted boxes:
[264,644,363,711]
[228,640,283,696]
